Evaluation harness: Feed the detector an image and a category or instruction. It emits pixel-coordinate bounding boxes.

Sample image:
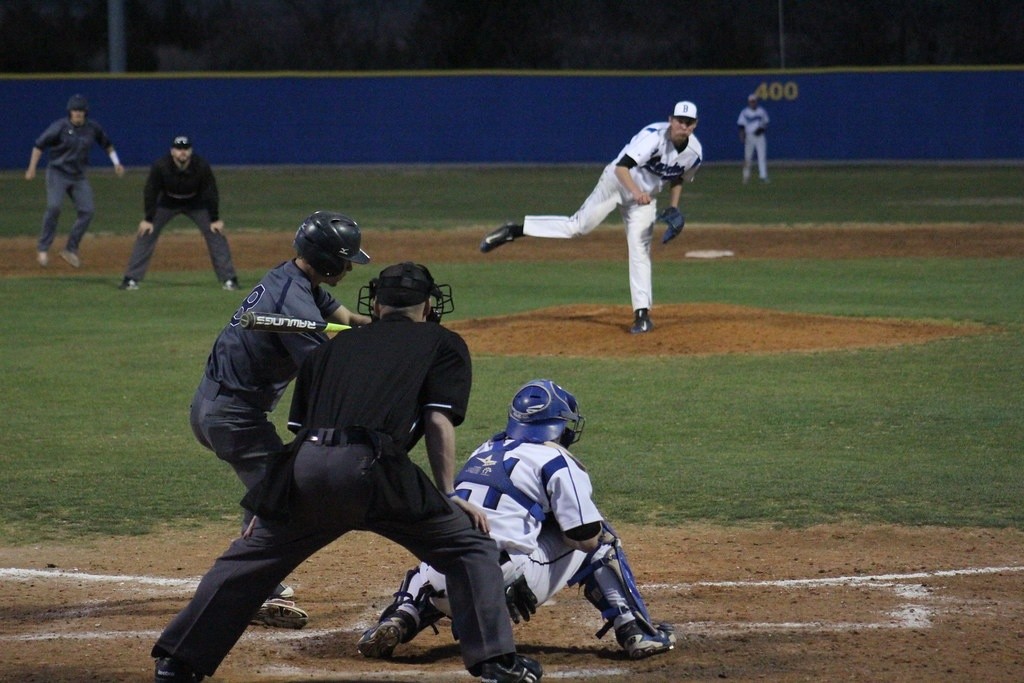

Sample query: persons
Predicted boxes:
[152,262,544,683]
[191,211,371,630]
[119,135,241,291]
[24,94,124,269]
[359,378,676,658]
[737,94,770,183]
[480,101,703,334]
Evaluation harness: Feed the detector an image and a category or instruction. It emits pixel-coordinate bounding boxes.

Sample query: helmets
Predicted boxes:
[505,380,575,442]
[291,210,371,276]
[68,93,90,112]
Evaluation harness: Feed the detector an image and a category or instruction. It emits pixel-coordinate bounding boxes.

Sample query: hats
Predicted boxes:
[376,263,430,307]
[171,136,191,149]
[748,95,755,100]
[672,100,697,119]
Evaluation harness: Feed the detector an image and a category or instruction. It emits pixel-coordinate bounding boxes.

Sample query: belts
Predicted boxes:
[498,549,511,567]
[304,429,368,444]
[218,386,232,396]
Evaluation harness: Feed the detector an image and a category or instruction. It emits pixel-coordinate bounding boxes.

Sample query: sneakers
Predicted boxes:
[357,617,407,658]
[253,600,308,628]
[481,655,542,683]
[631,309,652,333]
[480,222,515,252]
[272,583,294,597]
[154,656,191,683]
[615,621,665,658]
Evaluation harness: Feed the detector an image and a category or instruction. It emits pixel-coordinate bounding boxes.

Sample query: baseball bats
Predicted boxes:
[239,311,356,334]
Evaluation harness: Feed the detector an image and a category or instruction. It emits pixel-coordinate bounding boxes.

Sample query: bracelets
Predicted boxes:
[447,492,456,499]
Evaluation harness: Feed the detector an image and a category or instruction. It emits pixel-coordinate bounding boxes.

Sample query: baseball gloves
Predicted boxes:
[658,206,685,244]
[755,127,764,135]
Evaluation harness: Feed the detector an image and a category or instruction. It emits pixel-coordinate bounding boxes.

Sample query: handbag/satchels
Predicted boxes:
[240,439,303,521]
[362,432,452,524]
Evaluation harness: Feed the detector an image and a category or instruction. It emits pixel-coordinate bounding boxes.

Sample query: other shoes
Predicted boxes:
[120,279,139,291]
[222,278,240,291]
[37,251,47,266]
[61,249,79,267]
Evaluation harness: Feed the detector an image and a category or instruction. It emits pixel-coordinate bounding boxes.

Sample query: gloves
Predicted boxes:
[502,574,538,623]
